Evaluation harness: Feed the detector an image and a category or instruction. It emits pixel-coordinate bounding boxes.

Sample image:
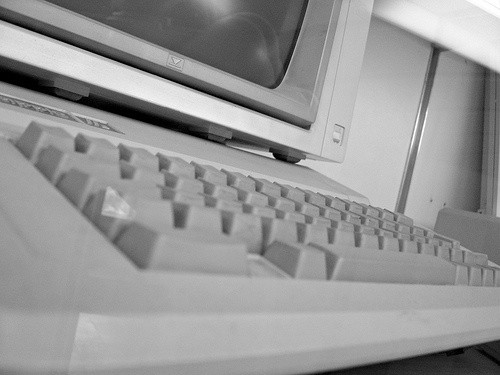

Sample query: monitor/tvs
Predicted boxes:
[0,0,377,166]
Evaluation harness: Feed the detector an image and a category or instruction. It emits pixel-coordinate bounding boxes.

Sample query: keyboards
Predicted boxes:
[1,119,500,374]
